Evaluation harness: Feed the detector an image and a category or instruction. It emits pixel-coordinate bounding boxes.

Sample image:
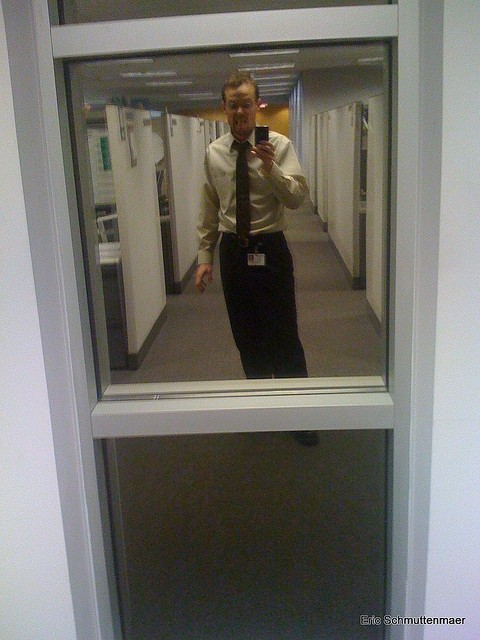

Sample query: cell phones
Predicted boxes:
[255,124,269,147]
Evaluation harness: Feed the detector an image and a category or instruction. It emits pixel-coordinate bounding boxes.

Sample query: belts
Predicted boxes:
[222,233,280,247]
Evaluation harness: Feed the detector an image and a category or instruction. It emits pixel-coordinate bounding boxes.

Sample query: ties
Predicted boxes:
[231,140,253,236]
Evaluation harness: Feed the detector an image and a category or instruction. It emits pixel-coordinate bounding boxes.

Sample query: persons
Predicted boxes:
[194,69,319,450]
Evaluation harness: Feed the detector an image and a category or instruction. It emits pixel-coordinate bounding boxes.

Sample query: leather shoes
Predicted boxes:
[292,432,320,447]
